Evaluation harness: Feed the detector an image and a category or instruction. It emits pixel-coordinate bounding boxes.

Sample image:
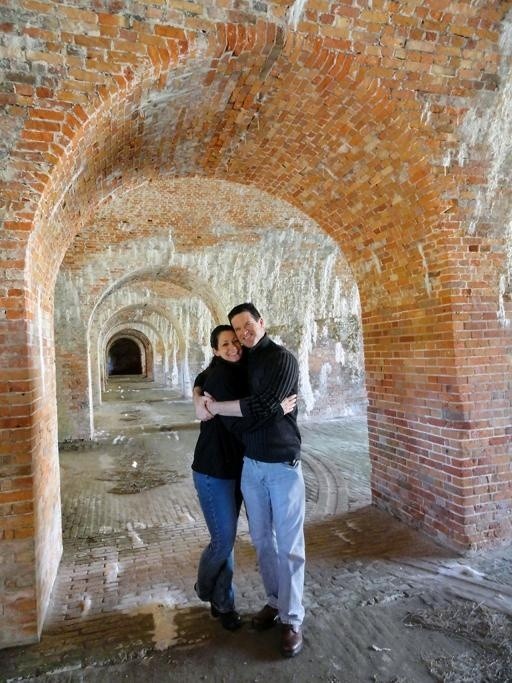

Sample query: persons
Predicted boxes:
[192,301,309,658]
[191,322,297,630]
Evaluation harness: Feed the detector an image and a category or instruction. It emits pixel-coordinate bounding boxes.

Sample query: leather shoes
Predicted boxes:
[251,604,280,631]
[280,623,304,658]
[211,604,242,631]
